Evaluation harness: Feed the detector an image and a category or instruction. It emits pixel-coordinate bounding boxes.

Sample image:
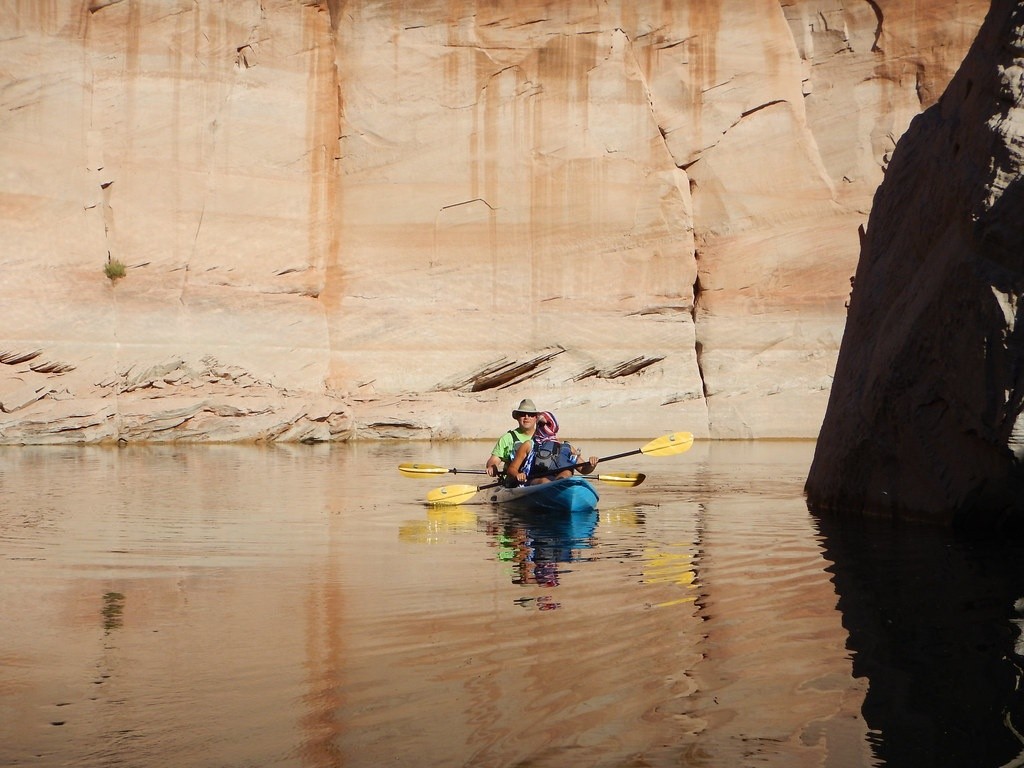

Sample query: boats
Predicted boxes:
[485,472,600,515]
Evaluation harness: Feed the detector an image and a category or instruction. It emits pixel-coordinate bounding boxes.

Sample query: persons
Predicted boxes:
[485,399,541,484]
[506,411,599,487]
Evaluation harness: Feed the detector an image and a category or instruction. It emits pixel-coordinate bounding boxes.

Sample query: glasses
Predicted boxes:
[538,421,551,428]
[518,412,536,417]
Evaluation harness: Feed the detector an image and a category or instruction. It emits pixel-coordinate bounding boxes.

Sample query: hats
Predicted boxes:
[512,399,540,419]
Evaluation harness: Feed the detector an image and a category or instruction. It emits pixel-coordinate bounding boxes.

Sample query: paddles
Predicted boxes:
[397,462,647,488]
[423,430,697,506]
